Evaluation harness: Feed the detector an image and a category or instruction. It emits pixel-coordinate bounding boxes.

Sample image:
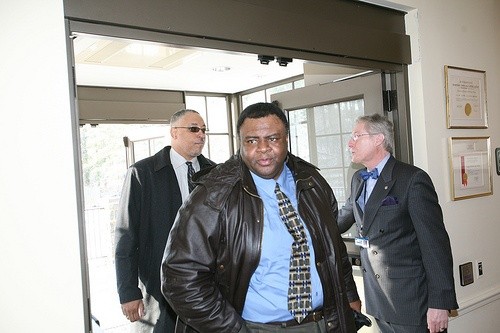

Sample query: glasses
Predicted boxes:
[174,126,209,132]
[351,132,385,142]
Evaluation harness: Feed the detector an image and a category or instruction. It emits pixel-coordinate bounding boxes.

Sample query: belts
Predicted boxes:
[279,311,326,326]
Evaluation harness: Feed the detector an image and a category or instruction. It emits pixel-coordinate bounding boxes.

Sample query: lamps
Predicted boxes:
[258,55,293,67]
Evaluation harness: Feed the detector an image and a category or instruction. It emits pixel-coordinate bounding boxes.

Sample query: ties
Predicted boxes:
[186,162,196,194]
[274,181,313,324]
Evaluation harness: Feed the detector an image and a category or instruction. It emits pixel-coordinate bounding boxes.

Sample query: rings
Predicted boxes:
[440,327,444,329]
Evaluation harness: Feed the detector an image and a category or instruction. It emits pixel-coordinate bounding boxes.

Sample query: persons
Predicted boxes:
[159,102,371,333]
[336,113,459,333]
[113,109,217,333]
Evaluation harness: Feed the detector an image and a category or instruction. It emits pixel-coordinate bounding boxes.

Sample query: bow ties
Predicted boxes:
[359,167,379,180]
